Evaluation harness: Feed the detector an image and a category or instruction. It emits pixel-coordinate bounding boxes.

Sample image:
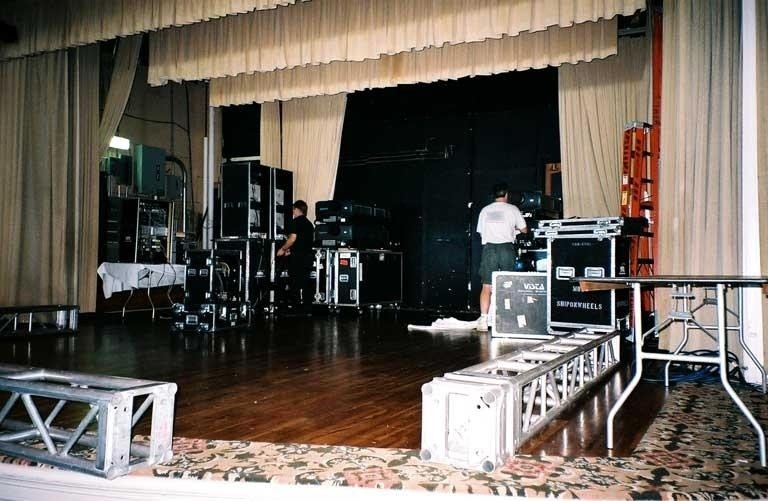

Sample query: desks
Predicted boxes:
[576,270,768,473]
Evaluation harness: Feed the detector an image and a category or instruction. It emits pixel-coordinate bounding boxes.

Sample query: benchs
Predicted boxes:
[1,304,80,336]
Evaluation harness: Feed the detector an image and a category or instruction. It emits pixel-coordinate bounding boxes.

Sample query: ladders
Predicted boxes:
[621,121,653,317]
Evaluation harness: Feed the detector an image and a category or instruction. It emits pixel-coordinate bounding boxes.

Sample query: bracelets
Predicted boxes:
[281,247,287,252]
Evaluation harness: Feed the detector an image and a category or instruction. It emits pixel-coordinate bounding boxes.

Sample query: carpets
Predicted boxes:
[0,375,768,500]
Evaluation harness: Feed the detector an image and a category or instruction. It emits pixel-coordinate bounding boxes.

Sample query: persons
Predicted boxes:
[277,200,315,316]
[476,187,528,332]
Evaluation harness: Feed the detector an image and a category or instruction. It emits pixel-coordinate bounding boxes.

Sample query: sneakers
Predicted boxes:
[477,316,489,332]
